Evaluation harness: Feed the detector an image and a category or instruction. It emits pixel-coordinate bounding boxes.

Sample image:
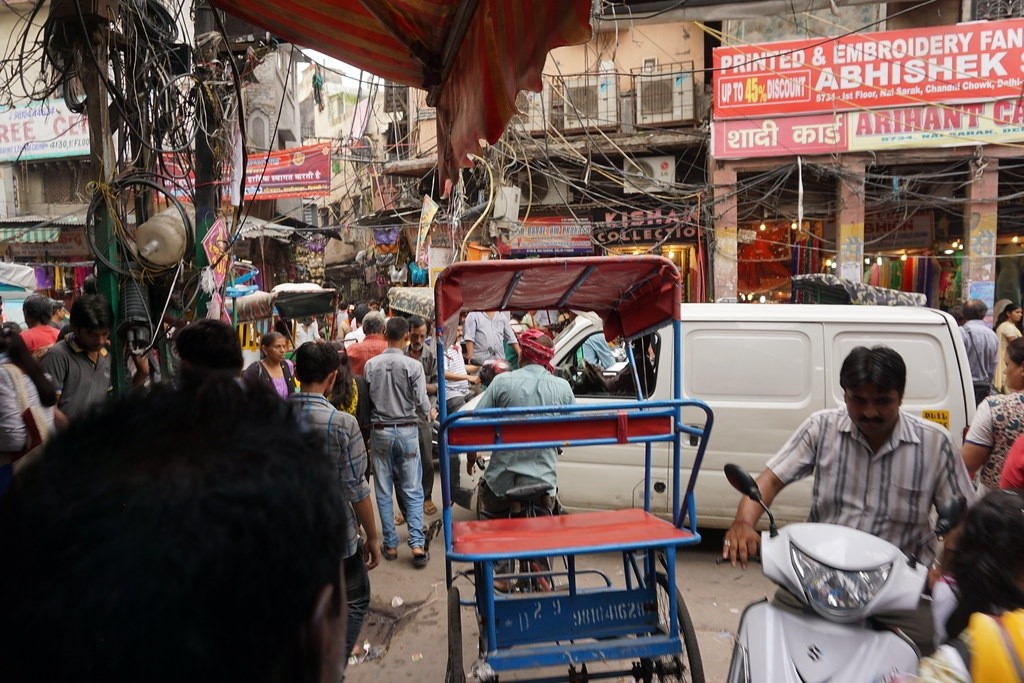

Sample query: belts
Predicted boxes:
[373,423,415,430]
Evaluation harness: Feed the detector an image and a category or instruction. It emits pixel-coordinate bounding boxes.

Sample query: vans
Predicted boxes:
[435,302,981,531]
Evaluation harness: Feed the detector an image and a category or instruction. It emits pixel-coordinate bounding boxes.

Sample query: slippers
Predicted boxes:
[411,547,430,566]
[380,543,397,560]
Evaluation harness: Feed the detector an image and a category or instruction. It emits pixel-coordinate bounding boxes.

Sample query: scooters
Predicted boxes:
[723,461,968,683]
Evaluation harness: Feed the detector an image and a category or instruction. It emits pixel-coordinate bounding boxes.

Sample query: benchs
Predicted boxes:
[438,399,714,563]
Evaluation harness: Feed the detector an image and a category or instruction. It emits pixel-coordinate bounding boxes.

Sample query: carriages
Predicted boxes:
[385,286,435,323]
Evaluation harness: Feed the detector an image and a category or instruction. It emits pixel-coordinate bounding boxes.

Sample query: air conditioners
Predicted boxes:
[564,79,620,130]
[507,84,552,132]
[636,69,694,124]
[518,170,570,205]
[623,155,676,194]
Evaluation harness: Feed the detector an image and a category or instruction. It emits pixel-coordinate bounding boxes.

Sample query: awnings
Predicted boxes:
[227,215,296,239]
[0,217,135,244]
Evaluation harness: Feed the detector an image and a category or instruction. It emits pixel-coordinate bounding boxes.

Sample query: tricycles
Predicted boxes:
[789,273,928,306]
[223,283,358,389]
[434,252,713,683]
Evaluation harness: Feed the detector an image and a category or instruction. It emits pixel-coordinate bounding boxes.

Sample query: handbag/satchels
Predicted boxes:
[3,364,48,453]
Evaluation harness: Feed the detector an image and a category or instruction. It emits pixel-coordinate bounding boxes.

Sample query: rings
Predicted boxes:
[724,540,730,547]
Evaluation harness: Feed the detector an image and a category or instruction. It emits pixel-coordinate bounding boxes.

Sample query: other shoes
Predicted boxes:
[395,512,405,526]
[493,579,510,593]
[424,500,437,515]
[530,562,551,593]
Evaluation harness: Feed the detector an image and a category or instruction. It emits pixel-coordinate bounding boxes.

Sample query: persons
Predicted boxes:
[960,338,1024,499]
[723,347,966,659]
[921,491,1023,683]
[949,306,967,330]
[0,289,619,683]
[590,335,652,397]
[958,299,998,408]
[992,304,1023,395]
[999,433,1024,495]
